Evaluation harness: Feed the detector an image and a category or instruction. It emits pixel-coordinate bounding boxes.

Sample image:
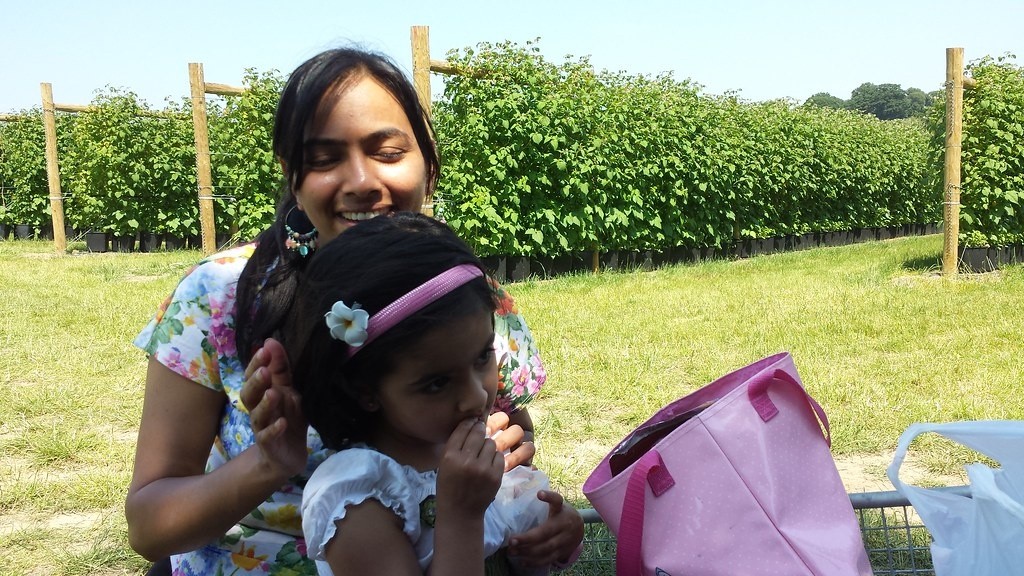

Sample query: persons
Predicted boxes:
[278,211,583,576]
[125,48,546,575]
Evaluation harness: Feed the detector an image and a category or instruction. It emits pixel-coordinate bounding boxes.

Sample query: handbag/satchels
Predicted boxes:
[885,417,1024,576]
[583,352,874,576]
